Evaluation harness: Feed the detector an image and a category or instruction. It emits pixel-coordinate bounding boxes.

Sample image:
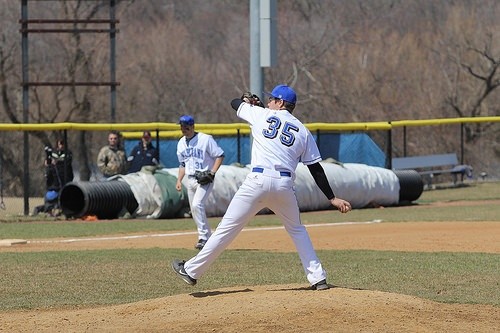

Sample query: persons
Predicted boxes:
[43,140,74,192]
[176,116,224,250]
[123,130,160,219]
[171,83,352,291]
[97,131,127,176]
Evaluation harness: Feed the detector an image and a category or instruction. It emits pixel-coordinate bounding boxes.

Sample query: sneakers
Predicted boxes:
[195,239,207,250]
[172,259,196,286]
[313,279,329,291]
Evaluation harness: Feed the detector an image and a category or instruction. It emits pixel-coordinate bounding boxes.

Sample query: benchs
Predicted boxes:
[392,152,474,190]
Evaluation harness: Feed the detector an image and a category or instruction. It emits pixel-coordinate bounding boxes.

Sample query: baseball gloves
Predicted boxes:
[241,91,265,108]
[195,169,215,186]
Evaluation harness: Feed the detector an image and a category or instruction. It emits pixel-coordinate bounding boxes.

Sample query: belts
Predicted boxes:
[252,168,290,177]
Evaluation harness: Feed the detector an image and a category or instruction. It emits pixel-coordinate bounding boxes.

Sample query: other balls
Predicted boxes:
[339,203,350,213]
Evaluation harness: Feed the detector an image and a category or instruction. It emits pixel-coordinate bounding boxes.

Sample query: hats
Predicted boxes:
[262,84,297,104]
[176,115,194,126]
